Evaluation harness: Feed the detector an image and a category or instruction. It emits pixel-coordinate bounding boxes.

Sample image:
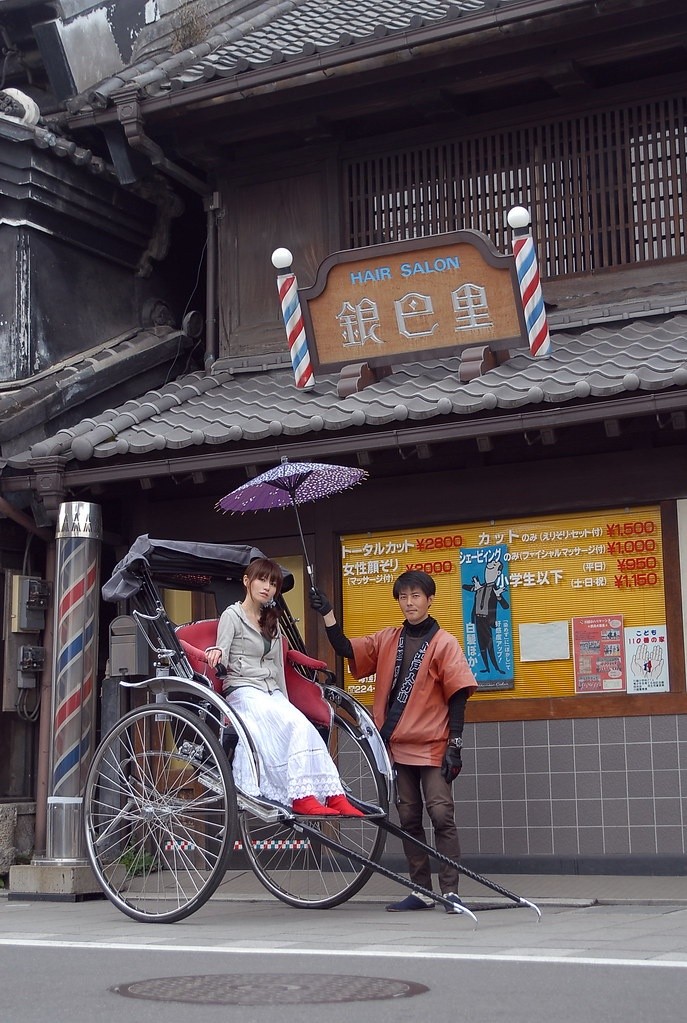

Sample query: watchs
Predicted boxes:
[447,738,464,748]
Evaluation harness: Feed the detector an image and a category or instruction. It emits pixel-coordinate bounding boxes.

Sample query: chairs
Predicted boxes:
[173,618,335,752]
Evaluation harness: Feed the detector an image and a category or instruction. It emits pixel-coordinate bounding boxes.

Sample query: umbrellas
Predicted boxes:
[214,456,369,594]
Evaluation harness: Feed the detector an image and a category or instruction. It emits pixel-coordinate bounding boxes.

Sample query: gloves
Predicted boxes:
[309,585,334,616]
[440,746,462,784]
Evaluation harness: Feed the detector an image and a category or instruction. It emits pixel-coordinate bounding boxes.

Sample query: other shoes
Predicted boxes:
[292,795,340,816]
[325,795,364,817]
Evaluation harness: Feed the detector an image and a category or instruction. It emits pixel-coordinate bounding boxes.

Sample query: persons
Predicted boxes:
[205,560,364,816]
[310,570,479,913]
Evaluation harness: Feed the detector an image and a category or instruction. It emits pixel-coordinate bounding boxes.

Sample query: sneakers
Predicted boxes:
[387,890,436,911]
[443,892,463,913]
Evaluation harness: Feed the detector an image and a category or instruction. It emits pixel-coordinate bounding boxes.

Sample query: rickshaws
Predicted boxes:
[80,534,542,928]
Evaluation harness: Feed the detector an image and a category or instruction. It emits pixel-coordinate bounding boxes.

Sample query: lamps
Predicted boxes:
[507,206,532,236]
[270,247,294,274]
